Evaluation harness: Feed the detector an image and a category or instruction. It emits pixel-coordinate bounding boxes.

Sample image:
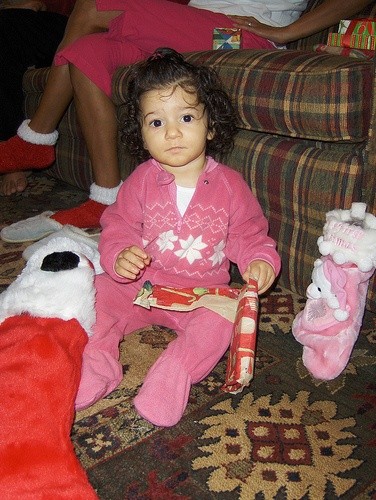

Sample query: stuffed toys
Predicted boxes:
[292,202,376,380]
[0,238,99,500]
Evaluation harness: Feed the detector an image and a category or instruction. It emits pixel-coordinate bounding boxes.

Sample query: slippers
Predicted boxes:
[5,206,55,241]
[22,220,101,263]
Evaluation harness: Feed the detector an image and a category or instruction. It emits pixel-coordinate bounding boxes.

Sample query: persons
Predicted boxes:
[74,47,281,427]
[0,0,371,230]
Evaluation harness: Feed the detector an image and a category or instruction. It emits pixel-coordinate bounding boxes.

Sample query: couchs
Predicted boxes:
[21,0,376,313]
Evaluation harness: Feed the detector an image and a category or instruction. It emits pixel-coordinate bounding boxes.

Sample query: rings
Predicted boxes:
[248,22,251,26]
